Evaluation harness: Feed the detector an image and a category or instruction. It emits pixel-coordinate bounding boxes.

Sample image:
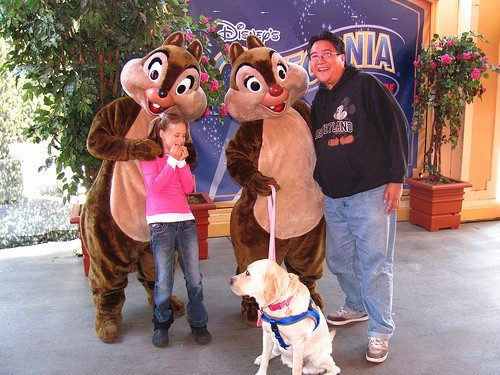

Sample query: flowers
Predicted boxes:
[0,0,231,206]
[411,30,500,183]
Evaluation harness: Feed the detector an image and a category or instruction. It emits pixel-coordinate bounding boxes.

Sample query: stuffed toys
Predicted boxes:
[224,35,326,326]
[79,31,208,343]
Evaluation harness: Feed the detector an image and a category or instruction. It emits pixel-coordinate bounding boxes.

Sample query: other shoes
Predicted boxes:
[193,330,212,345]
[152,328,169,346]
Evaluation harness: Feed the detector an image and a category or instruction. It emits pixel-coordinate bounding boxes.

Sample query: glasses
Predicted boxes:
[309,51,341,62]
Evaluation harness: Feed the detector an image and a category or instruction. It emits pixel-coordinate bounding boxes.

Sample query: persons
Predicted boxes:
[140,114,212,347]
[307,30,408,363]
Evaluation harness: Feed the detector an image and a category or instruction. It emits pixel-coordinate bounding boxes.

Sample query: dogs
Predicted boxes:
[228,259,341,375]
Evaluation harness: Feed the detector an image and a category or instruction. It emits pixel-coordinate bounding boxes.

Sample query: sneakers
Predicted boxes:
[365,337,389,362]
[327,306,369,325]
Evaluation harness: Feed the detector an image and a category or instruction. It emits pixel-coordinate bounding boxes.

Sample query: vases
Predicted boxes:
[69,191,216,277]
[406,177,473,233]
